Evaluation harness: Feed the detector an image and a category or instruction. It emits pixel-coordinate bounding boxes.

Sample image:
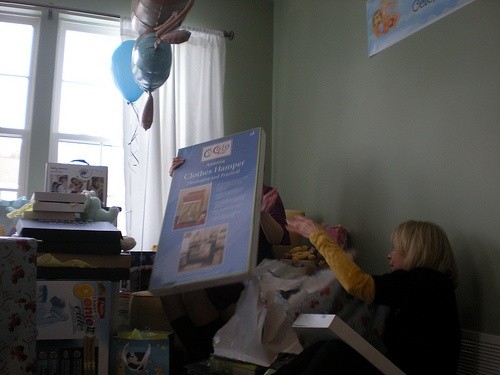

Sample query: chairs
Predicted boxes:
[125,210,306,328]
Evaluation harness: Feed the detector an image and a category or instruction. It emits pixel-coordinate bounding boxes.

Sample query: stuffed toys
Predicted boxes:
[81,189,119,224]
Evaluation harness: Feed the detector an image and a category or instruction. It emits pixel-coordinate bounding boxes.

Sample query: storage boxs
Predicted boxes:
[16,159,131,268]
[292,313,405,375]
[149,127,266,294]
[109,329,169,375]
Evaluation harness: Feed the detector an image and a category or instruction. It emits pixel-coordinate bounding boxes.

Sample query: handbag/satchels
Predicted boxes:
[112,329,174,375]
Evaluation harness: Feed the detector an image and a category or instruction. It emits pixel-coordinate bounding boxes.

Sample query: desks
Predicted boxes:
[34,266,130,375]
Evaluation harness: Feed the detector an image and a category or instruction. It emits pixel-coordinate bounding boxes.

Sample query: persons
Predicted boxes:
[273,215,456,375]
[160,156,290,363]
[51,176,99,194]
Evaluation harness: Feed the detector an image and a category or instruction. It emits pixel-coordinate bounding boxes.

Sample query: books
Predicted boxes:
[23,192,86,221]
